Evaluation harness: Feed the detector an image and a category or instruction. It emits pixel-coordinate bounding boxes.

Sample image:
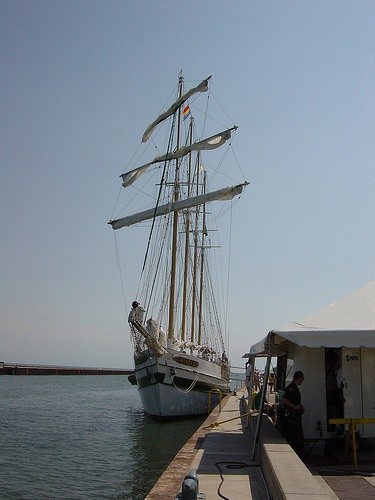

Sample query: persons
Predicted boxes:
[252,385,278,425]
[252,371,276,394]
[244,357,254,408]
[221,351,226,361]
[275,371,304,458]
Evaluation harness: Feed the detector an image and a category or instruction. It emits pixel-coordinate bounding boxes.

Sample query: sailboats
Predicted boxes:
[109,70,251,419]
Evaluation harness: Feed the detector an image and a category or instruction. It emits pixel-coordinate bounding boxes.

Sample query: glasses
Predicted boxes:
[302,378,304,380]
